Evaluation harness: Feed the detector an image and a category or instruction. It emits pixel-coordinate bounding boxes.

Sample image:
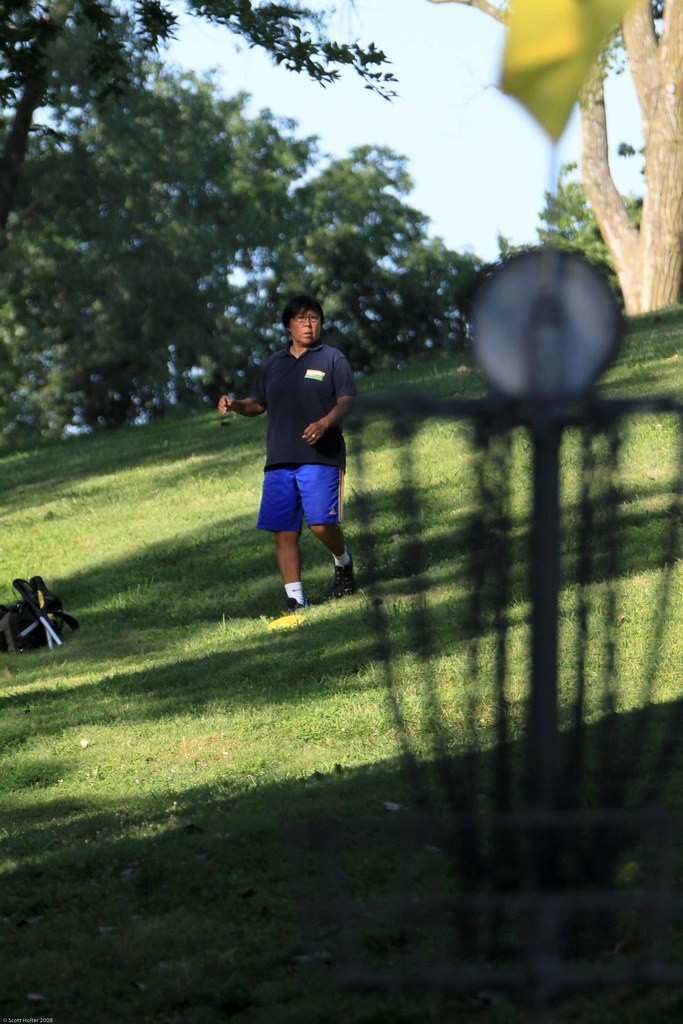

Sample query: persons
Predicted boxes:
[218,294,356,617]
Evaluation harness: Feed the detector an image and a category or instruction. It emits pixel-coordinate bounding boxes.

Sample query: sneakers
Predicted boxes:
[334,551,355,599]
[285,597,311,616]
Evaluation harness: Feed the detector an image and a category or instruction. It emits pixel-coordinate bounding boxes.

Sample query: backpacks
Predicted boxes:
[0,576,79,654]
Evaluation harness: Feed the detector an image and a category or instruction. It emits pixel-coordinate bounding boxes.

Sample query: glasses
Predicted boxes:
[290,312,321,323]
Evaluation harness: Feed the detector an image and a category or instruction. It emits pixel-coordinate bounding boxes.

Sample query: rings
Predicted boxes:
[312,434,315,438]
